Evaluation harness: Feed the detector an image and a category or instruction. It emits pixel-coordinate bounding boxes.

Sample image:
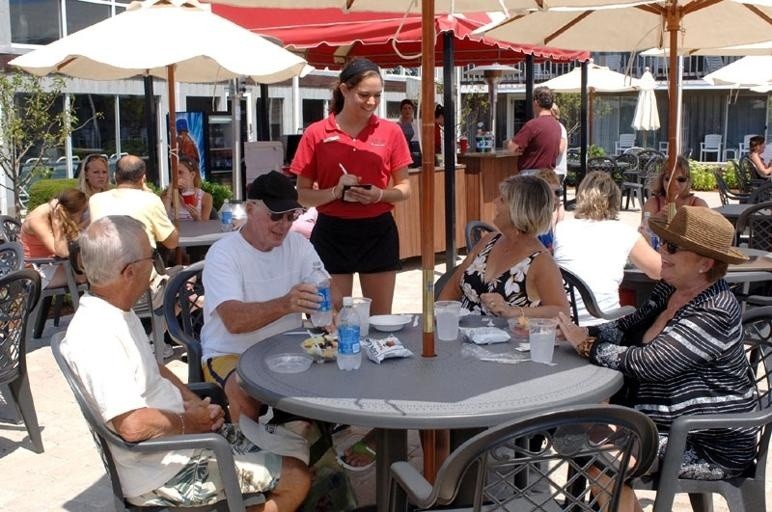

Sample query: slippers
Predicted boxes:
[338,441,376,474]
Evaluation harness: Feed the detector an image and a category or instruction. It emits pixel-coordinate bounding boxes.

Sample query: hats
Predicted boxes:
[648,205,750,265]
[246,170,304,214]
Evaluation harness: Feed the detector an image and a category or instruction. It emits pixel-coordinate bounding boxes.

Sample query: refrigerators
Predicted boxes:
[171,110,232,193]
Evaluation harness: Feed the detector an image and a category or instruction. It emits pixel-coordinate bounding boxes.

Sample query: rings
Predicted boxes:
[490,301,495,305]
[571,320,575,325]
[568,323,571,326]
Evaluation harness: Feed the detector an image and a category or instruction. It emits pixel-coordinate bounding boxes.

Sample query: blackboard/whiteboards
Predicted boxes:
[244,141,284,198]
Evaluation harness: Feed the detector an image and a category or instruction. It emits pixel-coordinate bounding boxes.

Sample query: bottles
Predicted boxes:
[485,130,491,151]
[177,119,199,180]
[309,258,334,328]
[475,129,483,150]
[460,137,468,152]
[642,210,657,250]
[336,296,364,370]
[222,198,235,231]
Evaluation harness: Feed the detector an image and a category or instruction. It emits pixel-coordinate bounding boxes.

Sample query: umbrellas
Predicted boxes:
[532,59,642,148]
[630,66,661,148]
[444,0,772,179]
[702,55,772,95]
[6,0,317,264]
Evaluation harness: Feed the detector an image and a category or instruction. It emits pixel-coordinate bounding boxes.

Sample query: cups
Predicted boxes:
[436,299,463,342]
[526,318,560,364]
[353,297,373,334]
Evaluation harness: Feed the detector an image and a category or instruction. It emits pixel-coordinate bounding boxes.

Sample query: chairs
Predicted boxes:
[1,199,771,512]
[560,134,771,213]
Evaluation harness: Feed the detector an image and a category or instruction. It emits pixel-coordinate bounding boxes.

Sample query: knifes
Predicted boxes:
[413,315,419,327]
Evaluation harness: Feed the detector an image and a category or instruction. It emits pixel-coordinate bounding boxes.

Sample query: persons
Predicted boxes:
[433,103,444,167]
[641,156,708,255]
[552,171,663,328]
[289,56,411,316]
[553,204,757,512]
[748,135,772,190]
[395,99,416,168]
[505,86,562,176]
[550,102,568,186]
[344,175,570,468]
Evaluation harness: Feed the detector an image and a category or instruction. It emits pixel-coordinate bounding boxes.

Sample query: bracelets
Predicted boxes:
[515,305,525,318]
[373,189,383,204]
[331,186,337,200]
[575,336,597,359]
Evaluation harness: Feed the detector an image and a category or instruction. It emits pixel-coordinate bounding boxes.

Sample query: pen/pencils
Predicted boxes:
[339,162,347,175]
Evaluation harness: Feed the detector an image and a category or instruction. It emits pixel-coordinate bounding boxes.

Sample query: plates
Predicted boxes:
[265,354,313,373]
[373,314,412,333]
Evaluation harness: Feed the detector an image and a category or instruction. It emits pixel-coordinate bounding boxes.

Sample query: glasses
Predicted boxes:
[659,236,691,255]
[251,200,300,222]
[121,250,158,273]
[665,175,688,182]
